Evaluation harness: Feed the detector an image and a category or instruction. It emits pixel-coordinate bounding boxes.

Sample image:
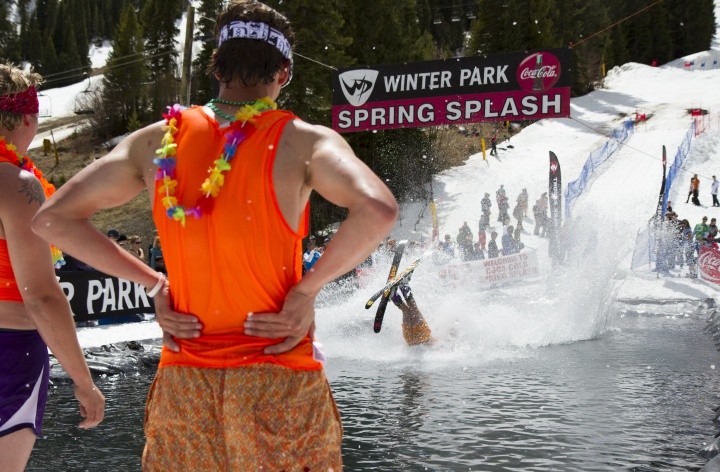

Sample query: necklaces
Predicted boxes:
[1,136,66,269]
[153,96,279,227]
[207,99,272,121]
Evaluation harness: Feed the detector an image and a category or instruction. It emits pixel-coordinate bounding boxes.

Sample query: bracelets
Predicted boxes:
[146,271,166,297]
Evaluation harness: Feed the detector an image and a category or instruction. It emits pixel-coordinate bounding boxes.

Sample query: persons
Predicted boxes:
[389,276,440,353]
[87,129,720,290]
[0,62,106,472]
[30,0,400,471]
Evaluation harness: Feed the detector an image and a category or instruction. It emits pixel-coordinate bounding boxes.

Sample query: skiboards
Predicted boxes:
[364,239,437,332]
